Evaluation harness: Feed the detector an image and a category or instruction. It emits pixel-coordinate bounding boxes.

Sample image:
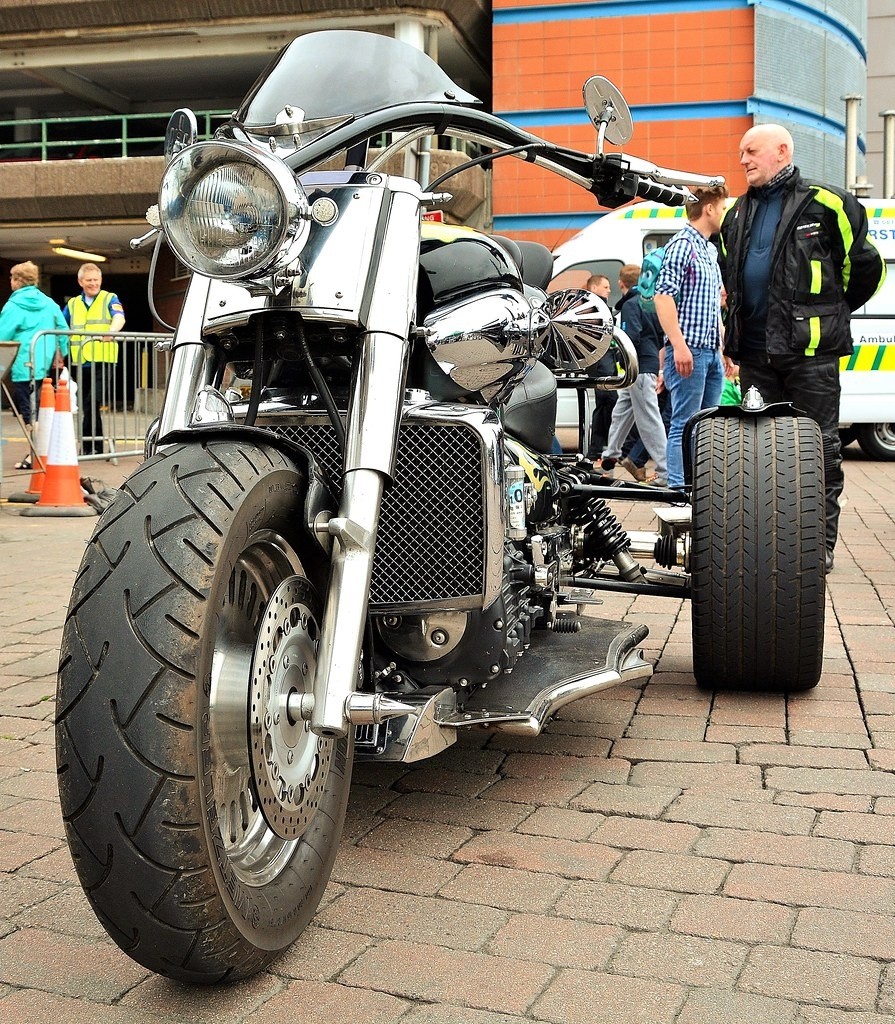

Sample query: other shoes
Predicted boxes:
[825,547,834,574]
[587,458,613,478]
[621,457,651,482]
[638,473,667,488]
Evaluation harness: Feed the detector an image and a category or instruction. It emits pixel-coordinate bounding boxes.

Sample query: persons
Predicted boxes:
[718,122,890,574]
[576,185,741,505]
[52,262,126,455]
[0,261,70,469]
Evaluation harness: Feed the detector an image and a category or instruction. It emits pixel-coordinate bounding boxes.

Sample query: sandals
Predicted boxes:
[14,454,33,469]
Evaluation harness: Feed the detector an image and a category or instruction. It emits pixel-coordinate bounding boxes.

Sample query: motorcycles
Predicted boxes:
[52,29,826,987]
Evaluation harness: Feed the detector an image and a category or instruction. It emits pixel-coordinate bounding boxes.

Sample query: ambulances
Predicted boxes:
[546,196,895,466]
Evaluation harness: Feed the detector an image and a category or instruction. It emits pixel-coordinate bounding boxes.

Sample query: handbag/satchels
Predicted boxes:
[637,237,692,313]
[60,366,79,415]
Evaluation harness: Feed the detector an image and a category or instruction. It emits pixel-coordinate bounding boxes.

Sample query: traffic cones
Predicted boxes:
[6,376,104,518]
